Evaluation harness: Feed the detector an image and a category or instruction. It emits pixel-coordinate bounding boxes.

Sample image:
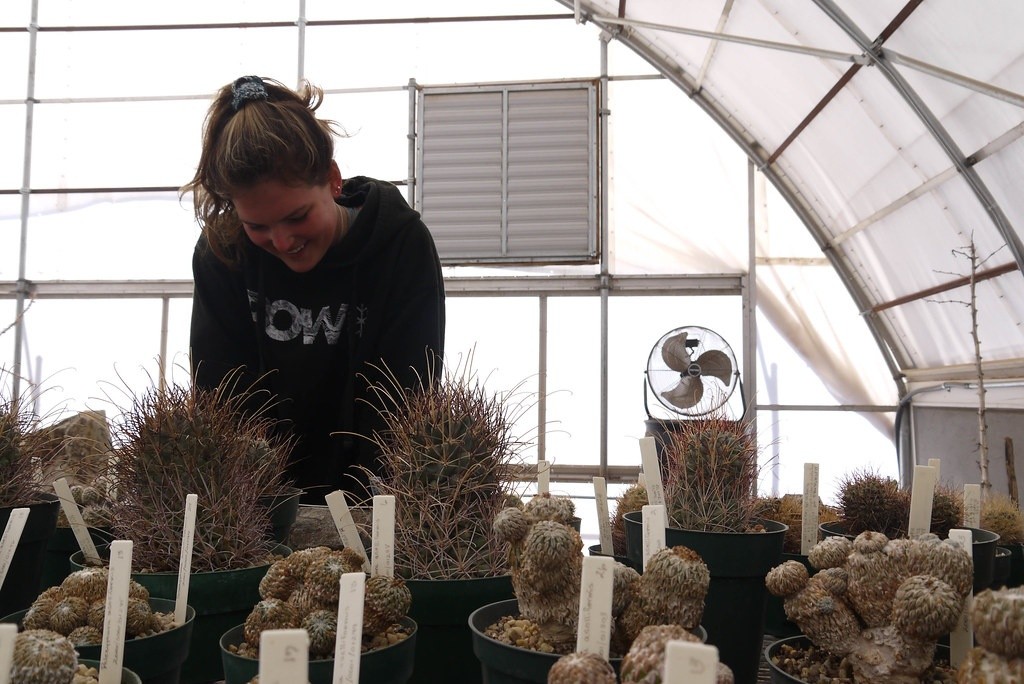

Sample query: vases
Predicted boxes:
[994,547,1011,589]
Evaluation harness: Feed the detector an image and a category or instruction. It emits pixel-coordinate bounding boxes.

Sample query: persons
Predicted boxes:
[188,74,446,504]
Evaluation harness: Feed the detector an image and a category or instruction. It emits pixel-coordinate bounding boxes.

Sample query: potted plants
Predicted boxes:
[622,413,788,684]
[69,542,293,684]
[468,506,710,684]
[818,468,908,542]
[221,547,418,684]
[984,497,1024,588]
[589,488,648,564]
[930,479,998,593]
[78,659,142,684]
[0,596,195,684]
[331,346,570,684]
[0,365,72,618]
[764,530,974,684]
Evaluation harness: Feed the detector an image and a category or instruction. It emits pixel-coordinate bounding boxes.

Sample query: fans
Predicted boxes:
[643,327,745,423]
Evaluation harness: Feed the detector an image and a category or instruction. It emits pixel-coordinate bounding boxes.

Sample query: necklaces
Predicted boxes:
[335,203,344,240]
